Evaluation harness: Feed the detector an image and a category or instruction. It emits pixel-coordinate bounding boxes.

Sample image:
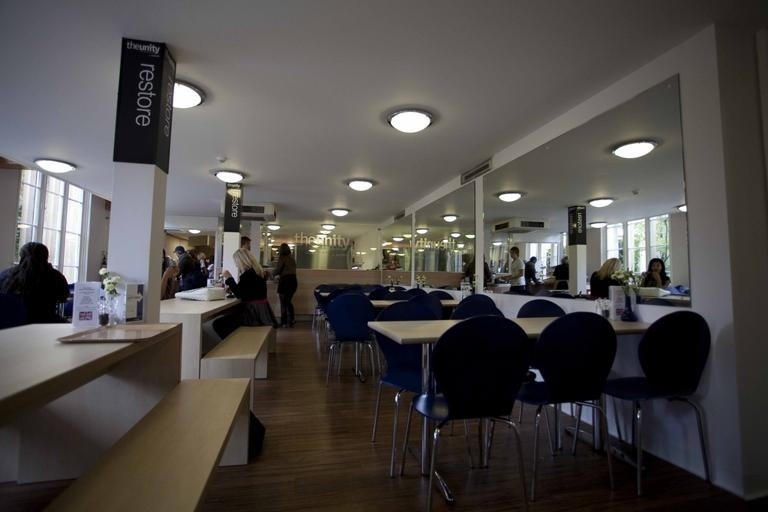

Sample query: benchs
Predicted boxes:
[197,326,274,413]
[43,378,251,511]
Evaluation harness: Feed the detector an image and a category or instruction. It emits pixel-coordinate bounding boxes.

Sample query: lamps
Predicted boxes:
[173,78,206,109]
[497,190,527,203]
[380,213,474,253]
[588,222,607,229]
[678,204,687,213]
[213,168,247,183]
[589,197,614,208]
[346,177,376,191]
[385,108,433,134]
[267,224,281,231]
[35,157,76,173]
[611,138,658,159]
[308,206,349,253]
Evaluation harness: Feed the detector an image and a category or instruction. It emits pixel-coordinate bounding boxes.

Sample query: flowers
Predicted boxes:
[98,267,121,297]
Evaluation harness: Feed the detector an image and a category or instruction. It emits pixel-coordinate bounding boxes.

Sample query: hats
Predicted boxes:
[172,245,185,255]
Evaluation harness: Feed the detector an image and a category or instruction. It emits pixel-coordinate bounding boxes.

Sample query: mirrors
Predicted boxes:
[378,213,411,273]
[414,180,475,275]
[482,72,694,309]
[260,218,378,271]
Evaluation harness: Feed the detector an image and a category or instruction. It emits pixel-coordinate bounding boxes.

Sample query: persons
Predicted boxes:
[589,258,625,299]
[0,242,70,333]
[640,258,671,289]
[159,235,300,329]
[465,246,569,294]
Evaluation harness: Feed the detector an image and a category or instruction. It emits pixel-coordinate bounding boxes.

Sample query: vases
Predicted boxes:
[107,296,119,326]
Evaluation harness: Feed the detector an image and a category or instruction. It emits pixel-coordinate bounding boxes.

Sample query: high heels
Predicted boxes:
[288,319,297,329]
[278,319,288,329]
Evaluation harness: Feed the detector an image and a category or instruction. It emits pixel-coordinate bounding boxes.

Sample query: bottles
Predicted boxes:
[97,296,108,326]
[206,277,214,288]
[595,298,611,319]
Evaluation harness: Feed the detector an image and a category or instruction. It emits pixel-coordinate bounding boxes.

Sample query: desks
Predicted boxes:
[158,293,242,380]
[0,322,182,486]
